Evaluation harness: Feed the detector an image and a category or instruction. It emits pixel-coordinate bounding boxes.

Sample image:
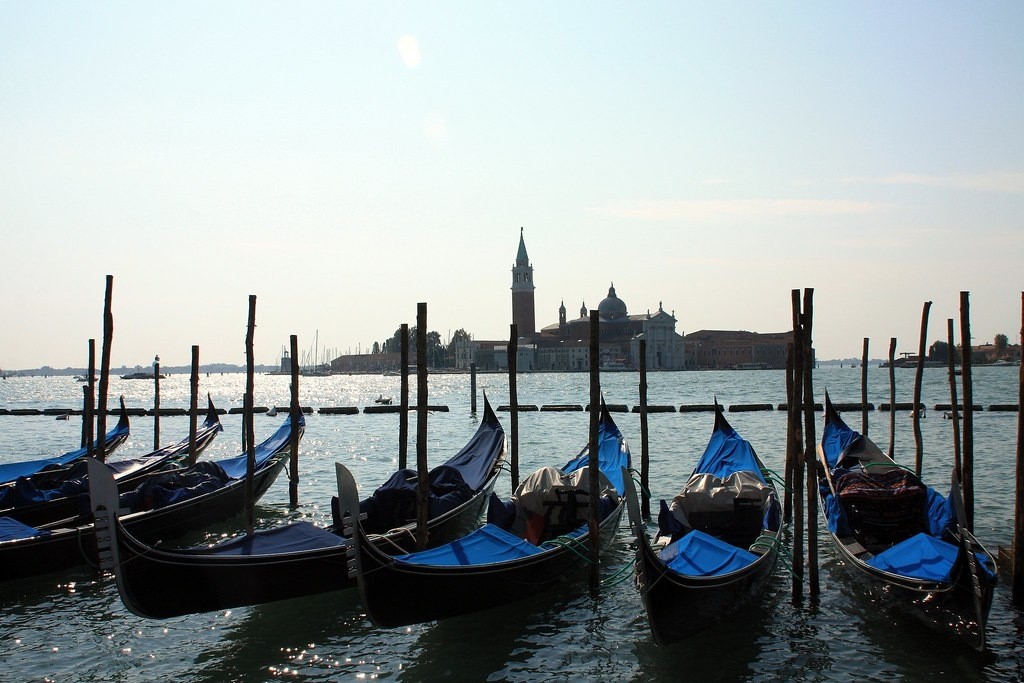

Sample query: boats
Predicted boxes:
[120,372,167,379]
[73,374,81,379]
[851,364,856,368]
[0,383,307,583]
[88,392,507,620]
[621,394,784,644]
[336,390,632,629]
[383,370,402,376]
[816,386,1000,653]
[946,366,973,375]
[77,371,99,382]
[302,329,332,377]
[264,359,279,375]
[985,360,1013,366]
[900,362,916,368]
[0,394,130,493]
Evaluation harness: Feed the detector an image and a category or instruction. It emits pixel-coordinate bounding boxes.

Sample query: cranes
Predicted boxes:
[900,352,916,358]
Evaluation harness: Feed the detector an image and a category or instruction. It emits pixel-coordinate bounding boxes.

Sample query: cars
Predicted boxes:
[469,362,480,371]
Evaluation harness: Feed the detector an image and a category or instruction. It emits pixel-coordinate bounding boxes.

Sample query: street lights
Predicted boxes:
[577,339,585,370]
[695,341,703,369]
[560,340,564,371]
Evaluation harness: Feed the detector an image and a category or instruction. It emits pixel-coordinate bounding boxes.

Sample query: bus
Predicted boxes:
[602,362,625,371]
